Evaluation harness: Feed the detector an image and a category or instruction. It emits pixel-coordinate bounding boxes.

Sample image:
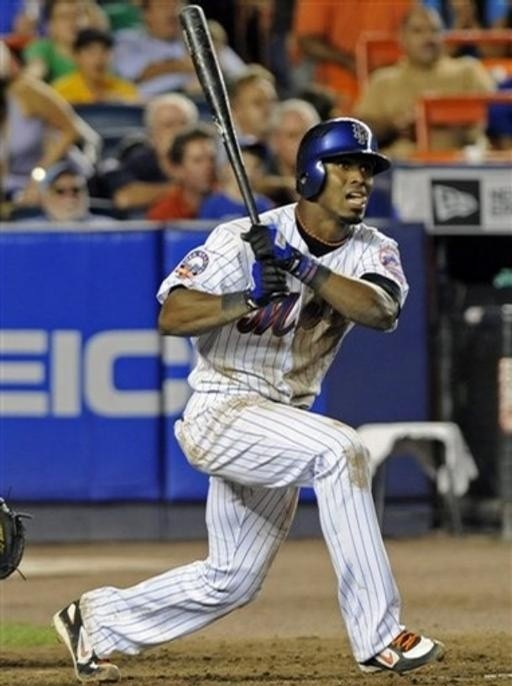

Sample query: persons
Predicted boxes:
[51,117,445,683]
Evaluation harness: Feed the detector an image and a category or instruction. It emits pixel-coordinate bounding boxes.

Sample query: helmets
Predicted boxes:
[290,115,391,204]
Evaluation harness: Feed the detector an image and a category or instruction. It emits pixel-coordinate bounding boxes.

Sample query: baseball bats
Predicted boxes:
[175,5,288,303]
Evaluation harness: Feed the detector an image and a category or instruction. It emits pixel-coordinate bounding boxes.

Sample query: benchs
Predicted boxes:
[360,30,512,86]
[412,96,512,163]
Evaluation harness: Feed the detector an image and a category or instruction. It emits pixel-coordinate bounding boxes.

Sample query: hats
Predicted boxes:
[42,160,88,188]
[73,26,114,48]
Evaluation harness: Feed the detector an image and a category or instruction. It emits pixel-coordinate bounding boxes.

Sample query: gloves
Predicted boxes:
[243,257,292,311]
[238,221,320,286]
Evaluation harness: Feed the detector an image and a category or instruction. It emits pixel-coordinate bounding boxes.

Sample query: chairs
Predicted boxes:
[11,100,146,223]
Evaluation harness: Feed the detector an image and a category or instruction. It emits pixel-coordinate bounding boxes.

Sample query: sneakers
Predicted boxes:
[52,598,122,683]
[355,630,448,674]
